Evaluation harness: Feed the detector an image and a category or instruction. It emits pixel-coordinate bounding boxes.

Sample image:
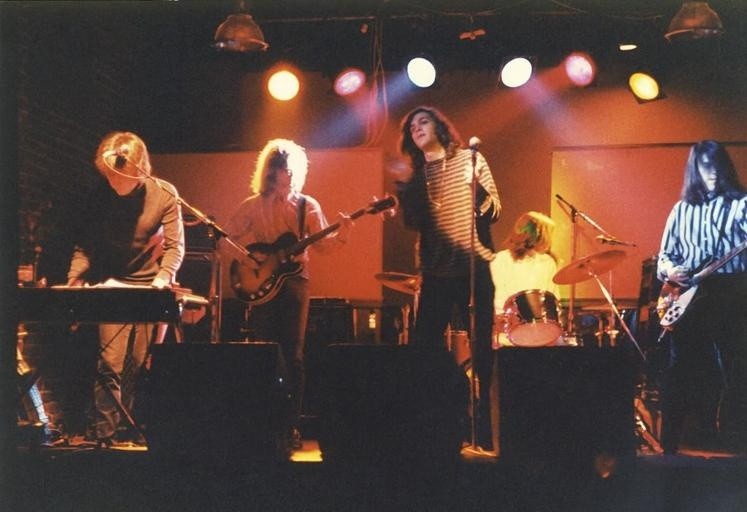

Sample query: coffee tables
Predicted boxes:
[374,272,422,295]
[579,305,639,311]
[553,249,627,285]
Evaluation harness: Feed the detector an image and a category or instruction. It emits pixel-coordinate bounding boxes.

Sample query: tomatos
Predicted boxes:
[20,287,210,447]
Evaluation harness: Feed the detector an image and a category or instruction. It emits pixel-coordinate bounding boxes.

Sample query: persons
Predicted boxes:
[490,208,563,315]
[398,105,501,444]
[656,138,746,439]
[211,134,356,441]
[63,133,188,438]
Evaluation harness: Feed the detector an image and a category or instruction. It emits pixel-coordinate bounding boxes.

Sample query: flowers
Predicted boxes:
[103,144,129,158]
[596,234,637,248]
[469,136,482,149]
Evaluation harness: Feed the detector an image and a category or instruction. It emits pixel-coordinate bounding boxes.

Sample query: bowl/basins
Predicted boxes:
[423,158,447,213]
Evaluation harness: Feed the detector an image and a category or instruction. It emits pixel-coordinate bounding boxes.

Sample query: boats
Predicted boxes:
[490,347,635,464]
[313,342,470,467]
[144,343,297,481]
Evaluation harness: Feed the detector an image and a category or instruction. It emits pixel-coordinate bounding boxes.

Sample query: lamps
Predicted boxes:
[328,56,365,96]
[627,66,668,102]
[266,68,300,102]
[407,52,444,88]
[494,53,537,88]
[209,0,269,52]
[565,49,608,87]
[459,24,486,40]
[665,2,726,44]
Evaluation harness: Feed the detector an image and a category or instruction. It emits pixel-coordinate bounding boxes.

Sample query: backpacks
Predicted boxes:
[592,328,623,350]
[502,289,563,346]
[638,255,662,305]
